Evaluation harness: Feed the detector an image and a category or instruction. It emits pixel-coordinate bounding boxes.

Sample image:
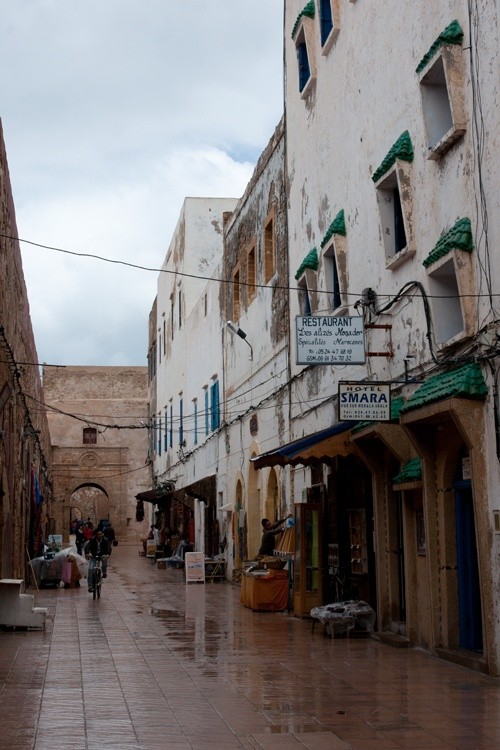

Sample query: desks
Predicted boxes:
[205,558,226,582]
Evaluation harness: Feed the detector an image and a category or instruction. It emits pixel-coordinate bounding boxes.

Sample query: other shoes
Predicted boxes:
[102,573,107,578]
[88,587,92,592]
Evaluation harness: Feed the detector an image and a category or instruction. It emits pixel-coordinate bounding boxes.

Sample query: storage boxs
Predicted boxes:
[157,559,182,569]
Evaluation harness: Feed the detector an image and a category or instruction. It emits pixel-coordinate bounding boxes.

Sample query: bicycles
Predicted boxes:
[85,554,110,601]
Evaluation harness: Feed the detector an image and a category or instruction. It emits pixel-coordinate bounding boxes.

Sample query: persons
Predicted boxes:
[84,528,113,592]
[70,517,116,557]
[259,514,292,556]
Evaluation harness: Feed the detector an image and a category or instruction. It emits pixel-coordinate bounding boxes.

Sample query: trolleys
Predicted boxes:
[157,546,184,569]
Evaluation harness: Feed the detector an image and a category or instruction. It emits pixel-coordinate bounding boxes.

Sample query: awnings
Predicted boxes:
[135,475,215,512]
